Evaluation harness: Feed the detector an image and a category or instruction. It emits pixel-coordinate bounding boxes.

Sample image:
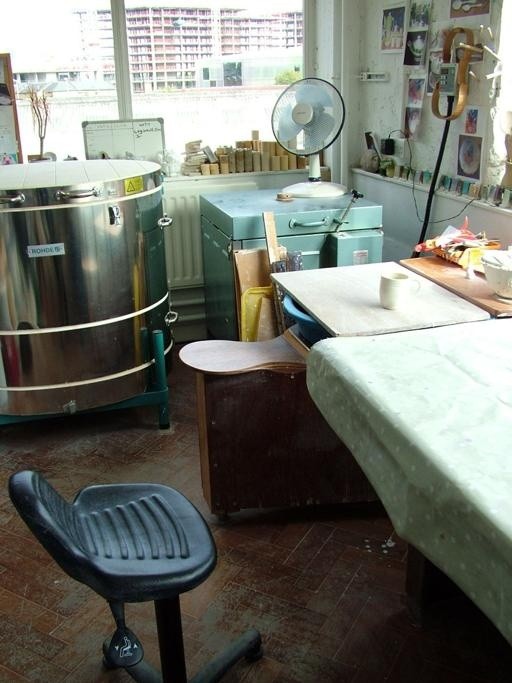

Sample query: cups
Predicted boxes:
[379,273,421,311]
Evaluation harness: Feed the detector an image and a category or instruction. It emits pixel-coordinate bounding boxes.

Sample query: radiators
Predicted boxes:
[162,182,259,292]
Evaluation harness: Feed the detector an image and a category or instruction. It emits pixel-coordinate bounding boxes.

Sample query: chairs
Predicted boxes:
[8,468,265,683]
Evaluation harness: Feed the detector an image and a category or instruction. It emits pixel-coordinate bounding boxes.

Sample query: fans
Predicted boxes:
[271,78,348,198]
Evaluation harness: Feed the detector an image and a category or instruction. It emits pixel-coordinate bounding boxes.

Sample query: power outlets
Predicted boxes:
[381,136,411,159]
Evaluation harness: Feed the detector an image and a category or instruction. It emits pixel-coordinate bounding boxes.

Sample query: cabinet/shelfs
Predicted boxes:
[179,326,380,522]
[306,317,512,650]
